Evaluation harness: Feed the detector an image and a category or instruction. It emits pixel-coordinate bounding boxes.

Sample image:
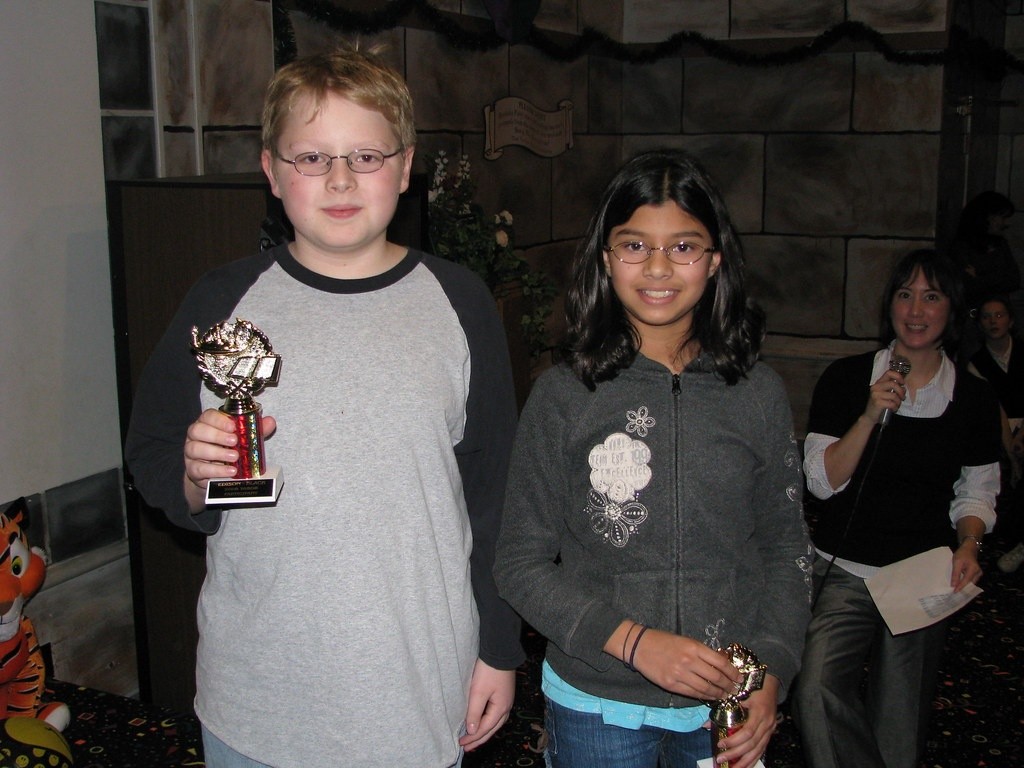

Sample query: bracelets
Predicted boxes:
[629,625,652,672]
[623,622,639,668]
[962,535,982,552]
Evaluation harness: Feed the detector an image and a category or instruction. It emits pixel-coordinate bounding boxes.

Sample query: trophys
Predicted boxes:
[188,318,283,506]
[696,641,766,768]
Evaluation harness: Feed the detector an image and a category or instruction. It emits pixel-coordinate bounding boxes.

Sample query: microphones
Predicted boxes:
[878,354,912,432]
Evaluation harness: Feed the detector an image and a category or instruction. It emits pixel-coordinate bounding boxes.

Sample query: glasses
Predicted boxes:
[603,241,714,265]
[276,145,405,176]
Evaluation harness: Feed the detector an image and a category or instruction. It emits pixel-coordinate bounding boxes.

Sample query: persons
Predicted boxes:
[491,149,815,768]
[954,191,1024,364]
[963,297,1024,572]
[792,248,1001,768]
[125,47,528,768]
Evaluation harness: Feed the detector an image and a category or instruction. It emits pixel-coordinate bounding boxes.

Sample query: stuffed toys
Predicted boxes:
[0,497,73,768]
